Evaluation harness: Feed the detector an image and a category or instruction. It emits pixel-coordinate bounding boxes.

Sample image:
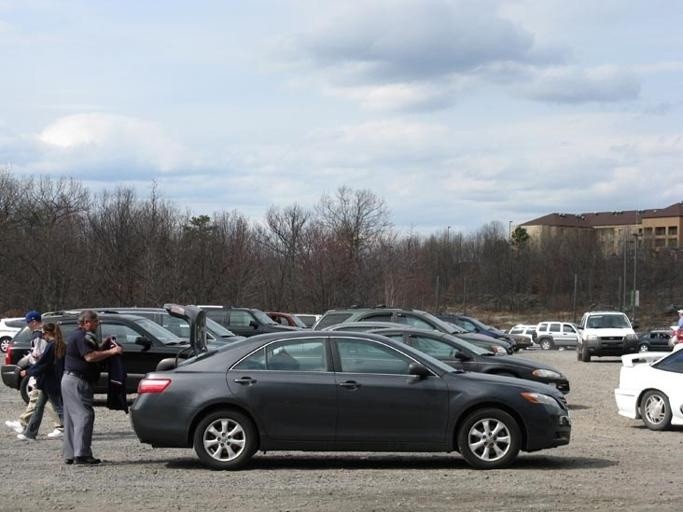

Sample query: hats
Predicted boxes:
[21,311,41,321]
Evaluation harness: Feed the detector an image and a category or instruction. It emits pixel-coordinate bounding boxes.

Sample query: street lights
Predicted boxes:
[446,225,451,242]
[631,231,643,326]
[506,219,513,242]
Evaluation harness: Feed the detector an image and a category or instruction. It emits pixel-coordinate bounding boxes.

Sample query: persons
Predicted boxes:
[16,322,65,440]
[58,308,121,464]
[3,310,63,438]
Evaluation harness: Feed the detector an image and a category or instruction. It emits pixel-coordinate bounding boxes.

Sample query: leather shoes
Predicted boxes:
[73,455,101,464]
[64,458,73,464]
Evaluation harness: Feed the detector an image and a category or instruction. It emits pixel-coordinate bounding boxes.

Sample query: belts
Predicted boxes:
[63,369,93,386]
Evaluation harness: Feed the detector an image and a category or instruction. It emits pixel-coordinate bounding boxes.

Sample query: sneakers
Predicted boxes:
[17,434,36,441]
[47,427,65,438]
[4,419,26,434]
